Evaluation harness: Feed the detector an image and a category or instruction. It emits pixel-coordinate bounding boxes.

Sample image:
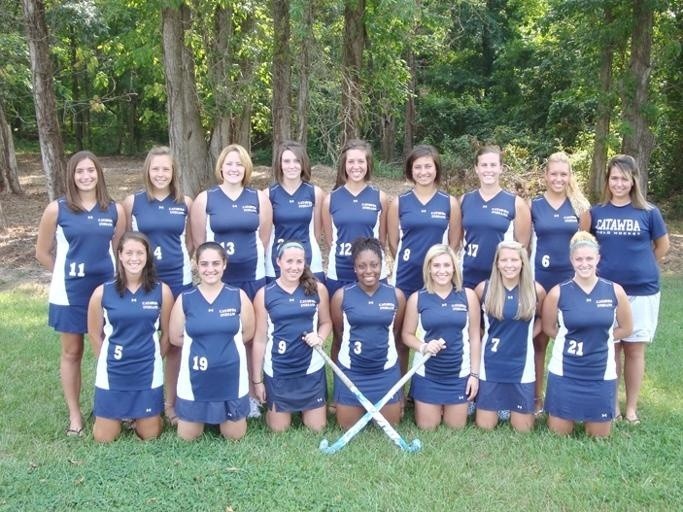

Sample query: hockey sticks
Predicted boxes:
[302,331,422,453]
[318,338,446,454]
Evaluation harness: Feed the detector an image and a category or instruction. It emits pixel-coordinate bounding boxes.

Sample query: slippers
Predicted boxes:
[67,425,86,439]
[614,413,640,426]
[164,415,177,426]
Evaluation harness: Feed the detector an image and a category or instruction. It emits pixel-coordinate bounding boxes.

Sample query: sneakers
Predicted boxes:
[467,400,476,416]
[248,397,262,418]
[497,410,511,421]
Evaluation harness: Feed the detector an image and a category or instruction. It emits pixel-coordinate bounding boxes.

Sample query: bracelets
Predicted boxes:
[470,372,480,379]
[252,379,264,384]
[420,343,426,353]
[318,336,324,341]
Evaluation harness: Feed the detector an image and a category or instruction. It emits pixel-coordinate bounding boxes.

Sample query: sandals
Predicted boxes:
[533,392,545,419]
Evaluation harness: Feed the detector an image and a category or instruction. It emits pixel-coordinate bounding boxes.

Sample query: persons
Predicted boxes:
[474,240,546,433]
[331,238,406,429]
[579,153,670,424]
[190,143,273,418]
[322,140,388,362]
[251,240,333,431]
[527,151,592,417]
[387,145,461,377]
[457,147,531,342]
[263,142,326,284]
[401,244,479,431]
[541,230,632,436]
[87,232,175,443]
[169,242,256,441]
[36,151,126,438]
[122,145,193,426]
